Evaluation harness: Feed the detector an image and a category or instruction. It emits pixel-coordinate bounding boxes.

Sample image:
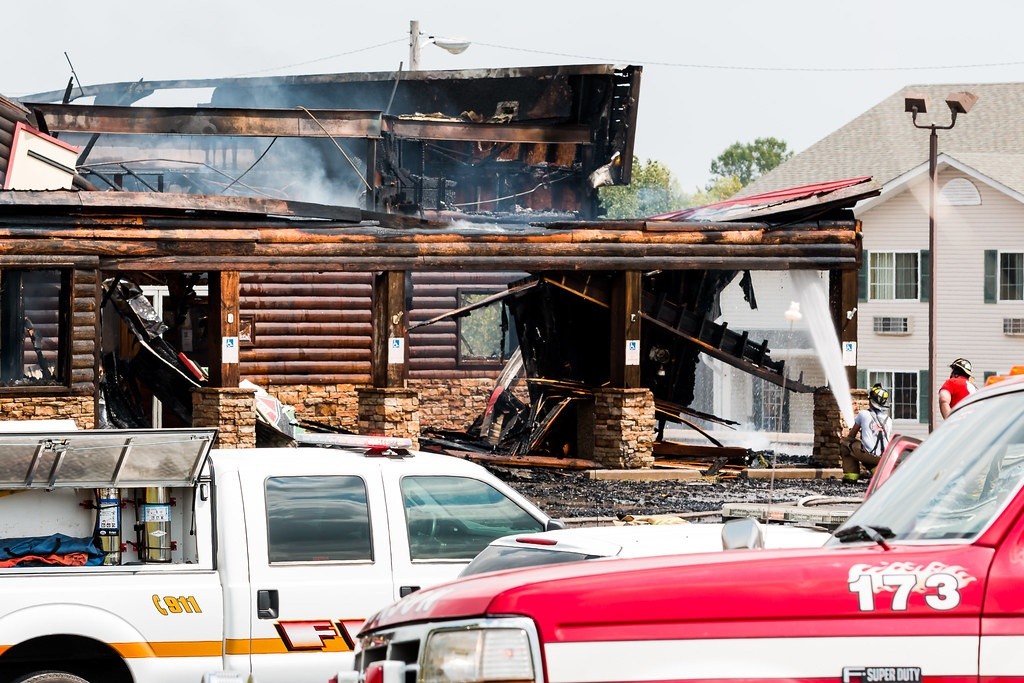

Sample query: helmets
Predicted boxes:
[869,383,890,411]
[950,358,975,379]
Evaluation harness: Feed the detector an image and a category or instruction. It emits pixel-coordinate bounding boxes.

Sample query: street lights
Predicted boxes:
[903,89,980,434]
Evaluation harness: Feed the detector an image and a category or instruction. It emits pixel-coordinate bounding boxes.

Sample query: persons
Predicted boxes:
[939,358,978,420]
[841,383,892,484]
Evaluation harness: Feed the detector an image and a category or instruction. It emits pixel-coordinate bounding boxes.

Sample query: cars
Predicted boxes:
[456,519,839,582]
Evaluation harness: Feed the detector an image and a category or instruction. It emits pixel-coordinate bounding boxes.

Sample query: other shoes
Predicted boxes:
[842,478,858,486]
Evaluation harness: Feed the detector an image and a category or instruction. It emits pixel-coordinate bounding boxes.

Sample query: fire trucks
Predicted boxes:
[327,370,1024,682]
[0,418,566,683]
